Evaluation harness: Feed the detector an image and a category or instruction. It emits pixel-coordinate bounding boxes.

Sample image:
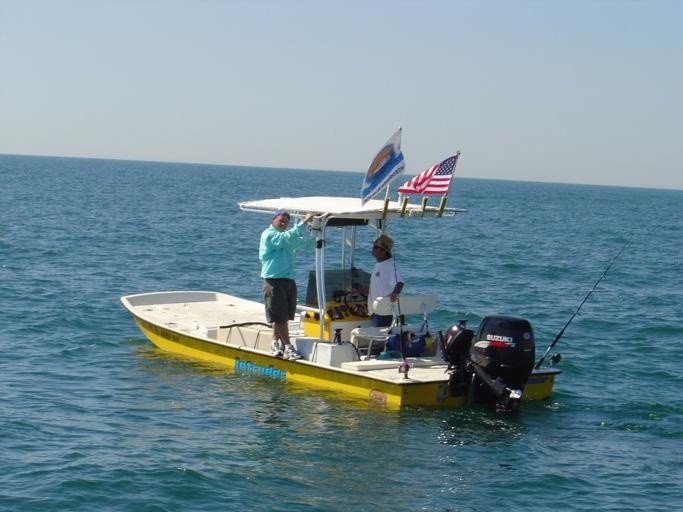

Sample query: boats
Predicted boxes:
[119,125,638,415]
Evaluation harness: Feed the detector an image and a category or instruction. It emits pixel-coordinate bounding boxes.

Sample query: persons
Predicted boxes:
[258,208,317,362]
[343,234,405,357]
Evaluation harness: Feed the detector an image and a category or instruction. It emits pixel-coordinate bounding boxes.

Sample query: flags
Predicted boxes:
[360,127,407,206]
[394,154,458,195]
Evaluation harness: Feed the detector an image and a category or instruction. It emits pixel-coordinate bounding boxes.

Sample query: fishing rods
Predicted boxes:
[392,252,409,378]
[535,236,635,369]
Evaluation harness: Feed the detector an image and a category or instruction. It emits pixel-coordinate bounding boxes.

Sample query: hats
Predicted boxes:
[368,235,394,253]
[273,208,290,221]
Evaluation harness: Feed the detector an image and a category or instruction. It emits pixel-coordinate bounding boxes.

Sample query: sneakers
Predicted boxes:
[282,346,303,361]
[270,343,284,357]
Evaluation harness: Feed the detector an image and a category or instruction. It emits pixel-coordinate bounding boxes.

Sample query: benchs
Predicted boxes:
[350,294,438,361]
